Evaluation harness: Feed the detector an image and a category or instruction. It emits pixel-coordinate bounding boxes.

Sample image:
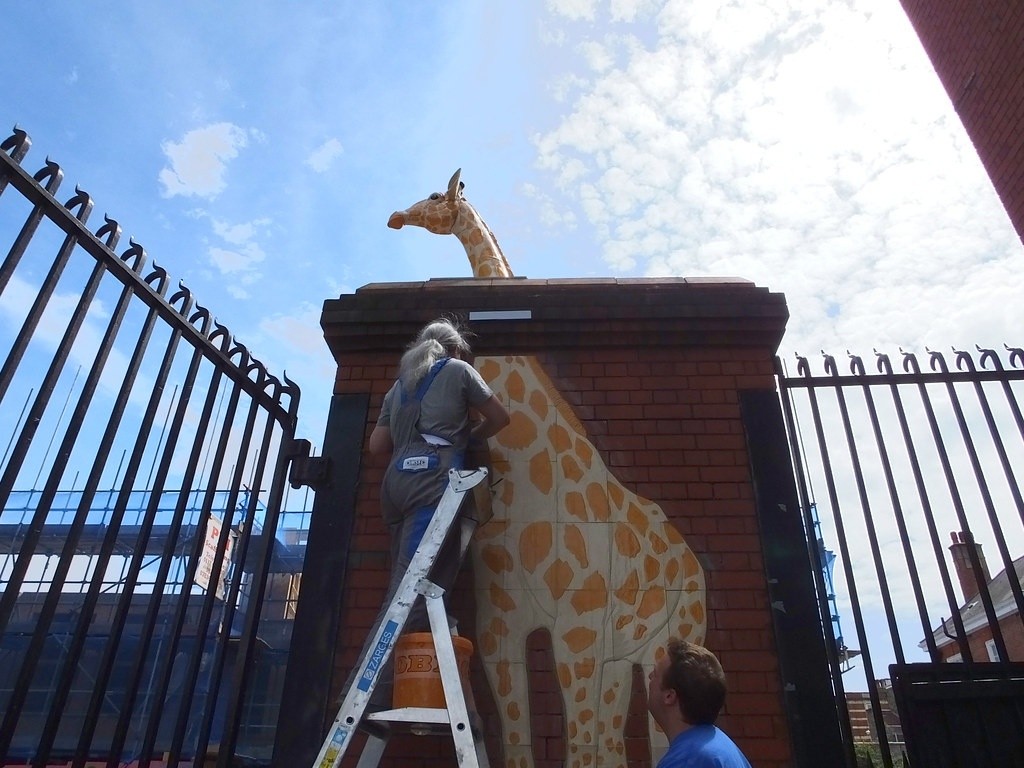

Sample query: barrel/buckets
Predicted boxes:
[392,633,473,710]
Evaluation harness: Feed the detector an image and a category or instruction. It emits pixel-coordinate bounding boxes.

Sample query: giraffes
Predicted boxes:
[385,168,511,279]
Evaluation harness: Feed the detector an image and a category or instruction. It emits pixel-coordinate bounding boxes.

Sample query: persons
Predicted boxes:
[647,637,753,768]
[336,320,511,733]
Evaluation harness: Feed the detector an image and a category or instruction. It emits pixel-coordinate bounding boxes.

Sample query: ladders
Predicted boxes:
[312,466,494,768]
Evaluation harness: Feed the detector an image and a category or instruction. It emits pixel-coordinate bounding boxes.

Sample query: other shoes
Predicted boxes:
[333,694,391,739]
